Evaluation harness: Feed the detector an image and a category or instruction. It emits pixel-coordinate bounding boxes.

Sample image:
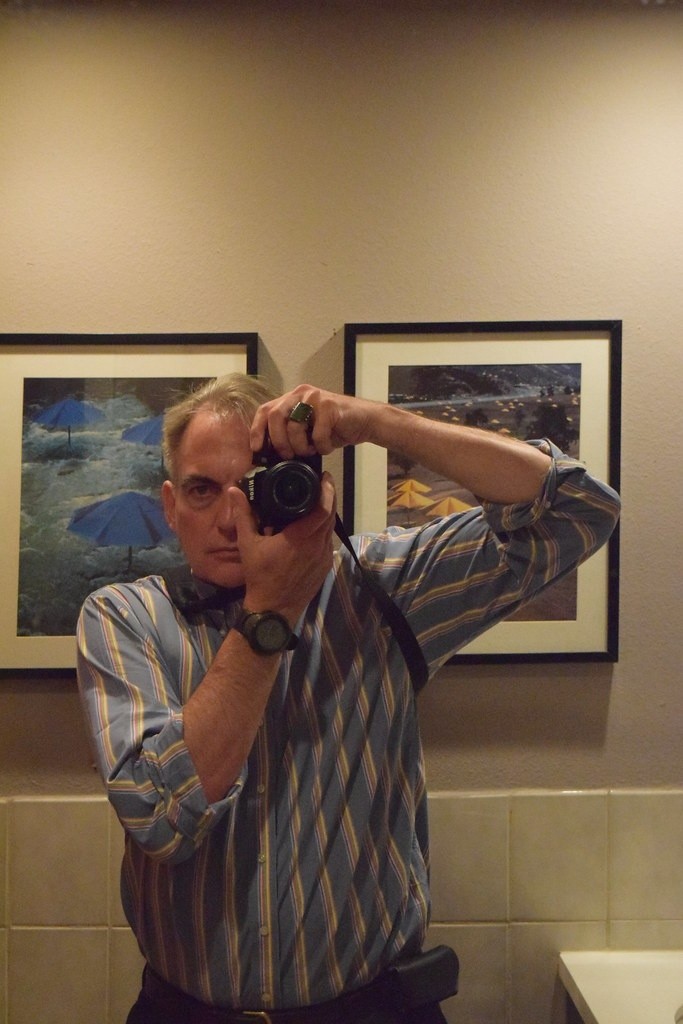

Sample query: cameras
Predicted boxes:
[238,434,323,535]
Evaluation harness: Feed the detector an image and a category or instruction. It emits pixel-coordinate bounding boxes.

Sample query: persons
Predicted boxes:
[75,372,620,1024]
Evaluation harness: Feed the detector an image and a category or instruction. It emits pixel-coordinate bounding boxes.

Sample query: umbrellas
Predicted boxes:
[387,478,473,527]
[121,413,165,471]
[67,491,175,572]
[33,399,102,445]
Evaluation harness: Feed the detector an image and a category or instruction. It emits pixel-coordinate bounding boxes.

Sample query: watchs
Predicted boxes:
[234,609,298,656]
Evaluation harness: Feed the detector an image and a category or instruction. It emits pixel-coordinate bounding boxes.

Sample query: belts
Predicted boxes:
[143,969,396,1024]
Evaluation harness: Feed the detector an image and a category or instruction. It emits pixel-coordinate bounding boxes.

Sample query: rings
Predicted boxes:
[290,401,314,422]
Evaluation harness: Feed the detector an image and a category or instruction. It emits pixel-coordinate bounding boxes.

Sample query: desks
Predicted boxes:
[557,949,683,1024]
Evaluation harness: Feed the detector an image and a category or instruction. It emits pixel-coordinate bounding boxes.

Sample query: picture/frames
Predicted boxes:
[343,320,624,665]
[0,333,259,680]
